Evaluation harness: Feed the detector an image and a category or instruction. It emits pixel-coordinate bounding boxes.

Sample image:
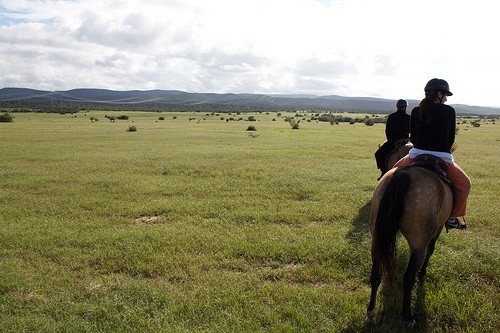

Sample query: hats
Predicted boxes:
[396,99,408,107]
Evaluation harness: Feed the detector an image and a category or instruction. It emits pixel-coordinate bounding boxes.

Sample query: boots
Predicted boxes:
[377,168,387,181]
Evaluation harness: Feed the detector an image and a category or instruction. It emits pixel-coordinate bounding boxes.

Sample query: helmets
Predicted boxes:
[424,78,453,96]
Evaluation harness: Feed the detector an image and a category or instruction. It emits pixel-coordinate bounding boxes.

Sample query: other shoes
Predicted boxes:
[445,218,466,230]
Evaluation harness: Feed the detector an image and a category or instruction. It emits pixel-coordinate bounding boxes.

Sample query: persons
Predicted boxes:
[393,78,470,229]
[374,99,411,180]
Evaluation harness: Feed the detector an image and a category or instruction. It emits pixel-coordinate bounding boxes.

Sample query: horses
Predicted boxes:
[384,141,413,172]
[364,165,454,326]
